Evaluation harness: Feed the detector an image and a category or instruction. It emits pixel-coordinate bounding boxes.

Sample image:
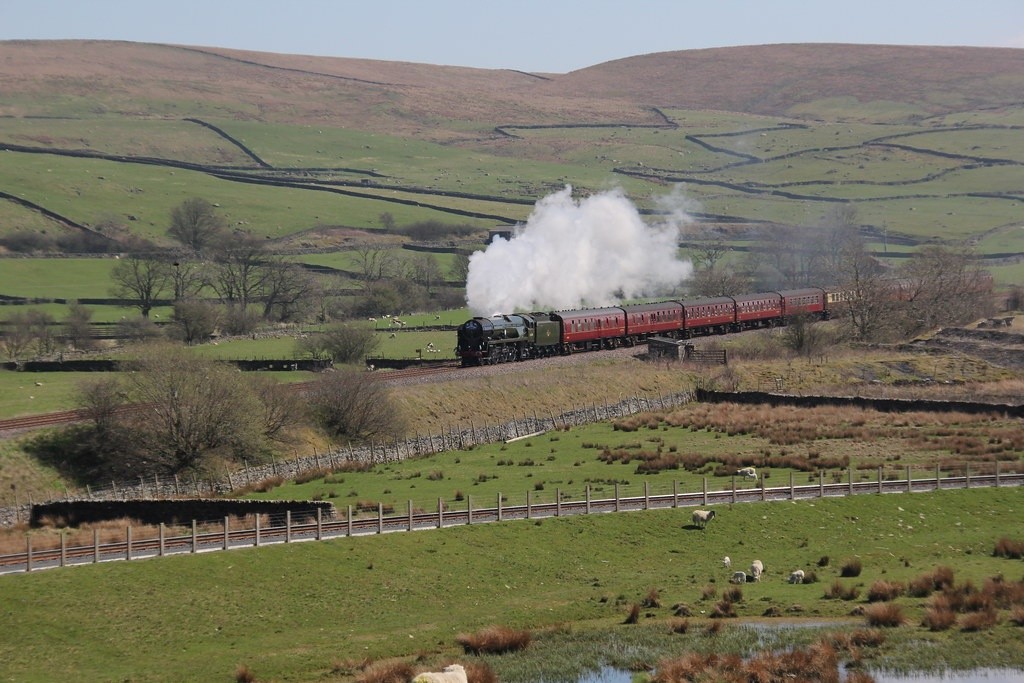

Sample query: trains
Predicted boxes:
[453,269,994,367]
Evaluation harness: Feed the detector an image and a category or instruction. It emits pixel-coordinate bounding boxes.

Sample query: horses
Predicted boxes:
[115,391,128,399]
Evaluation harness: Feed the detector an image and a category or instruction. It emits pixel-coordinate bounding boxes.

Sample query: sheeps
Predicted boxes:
[18,381,42,399]
[364,311,444,372]
[722,556,731,569]
[692,510,716,527]
[789,570,804,584]
[730,571,746,583]
[751,560,763,583]
[737,466,757,481]
